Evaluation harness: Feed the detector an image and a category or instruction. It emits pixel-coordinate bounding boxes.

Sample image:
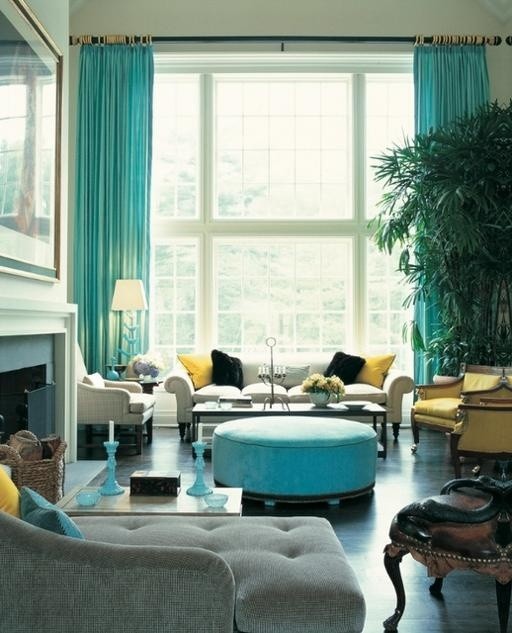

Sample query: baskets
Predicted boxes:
[1,430,69,505]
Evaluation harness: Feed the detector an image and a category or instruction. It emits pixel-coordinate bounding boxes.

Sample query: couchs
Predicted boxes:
[0,517,369,628]
[212,415,379,510]
[413,363,512,456]
[163,351,413,445]
[76,343,154,456]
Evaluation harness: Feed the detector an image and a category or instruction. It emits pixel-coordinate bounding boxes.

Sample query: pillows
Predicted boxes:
[177,351,212,392]
[327,351,365,380]
[355,354,395,384]
[211,347,243,386]
[21,484,88,539]
[0,468,24,517]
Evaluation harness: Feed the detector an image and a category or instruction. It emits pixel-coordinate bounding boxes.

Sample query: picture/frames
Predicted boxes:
[3,1,68,285]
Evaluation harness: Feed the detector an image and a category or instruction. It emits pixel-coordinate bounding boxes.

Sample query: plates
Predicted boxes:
[343,404,368,409]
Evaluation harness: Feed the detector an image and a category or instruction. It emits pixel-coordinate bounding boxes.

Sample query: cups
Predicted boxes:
[76,491,104,507]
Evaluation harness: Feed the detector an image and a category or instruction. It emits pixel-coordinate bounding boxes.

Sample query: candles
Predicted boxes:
[101,419,123,495]
[188,422,213,496]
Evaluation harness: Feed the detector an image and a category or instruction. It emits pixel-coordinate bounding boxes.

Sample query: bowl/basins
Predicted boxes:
[205,494,228,508]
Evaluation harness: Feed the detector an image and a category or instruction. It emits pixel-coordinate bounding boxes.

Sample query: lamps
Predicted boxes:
[112,279,153,379]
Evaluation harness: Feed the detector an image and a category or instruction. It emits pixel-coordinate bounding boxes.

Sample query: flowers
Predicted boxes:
[300,374,347,394]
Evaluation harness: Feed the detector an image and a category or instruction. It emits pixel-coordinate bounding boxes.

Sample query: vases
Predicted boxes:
[311,391,335,409]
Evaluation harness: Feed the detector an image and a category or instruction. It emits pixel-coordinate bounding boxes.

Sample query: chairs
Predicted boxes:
[382,474,511,629]
